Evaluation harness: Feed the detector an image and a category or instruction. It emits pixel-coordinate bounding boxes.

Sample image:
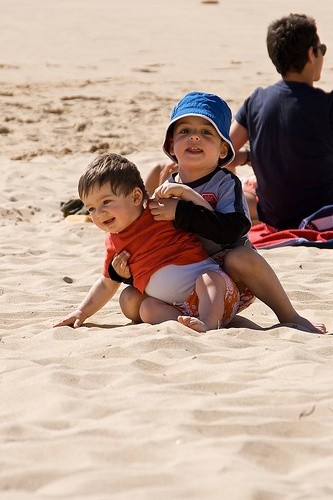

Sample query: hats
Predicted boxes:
[162,92,235,168]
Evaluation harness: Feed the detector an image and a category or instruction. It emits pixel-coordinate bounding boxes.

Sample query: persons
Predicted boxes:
[229,13,333,232]
[107,92,328,334]
[53,154,240,332]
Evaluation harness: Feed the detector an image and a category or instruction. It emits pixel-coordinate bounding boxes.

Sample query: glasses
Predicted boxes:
[313,44,326,56]
